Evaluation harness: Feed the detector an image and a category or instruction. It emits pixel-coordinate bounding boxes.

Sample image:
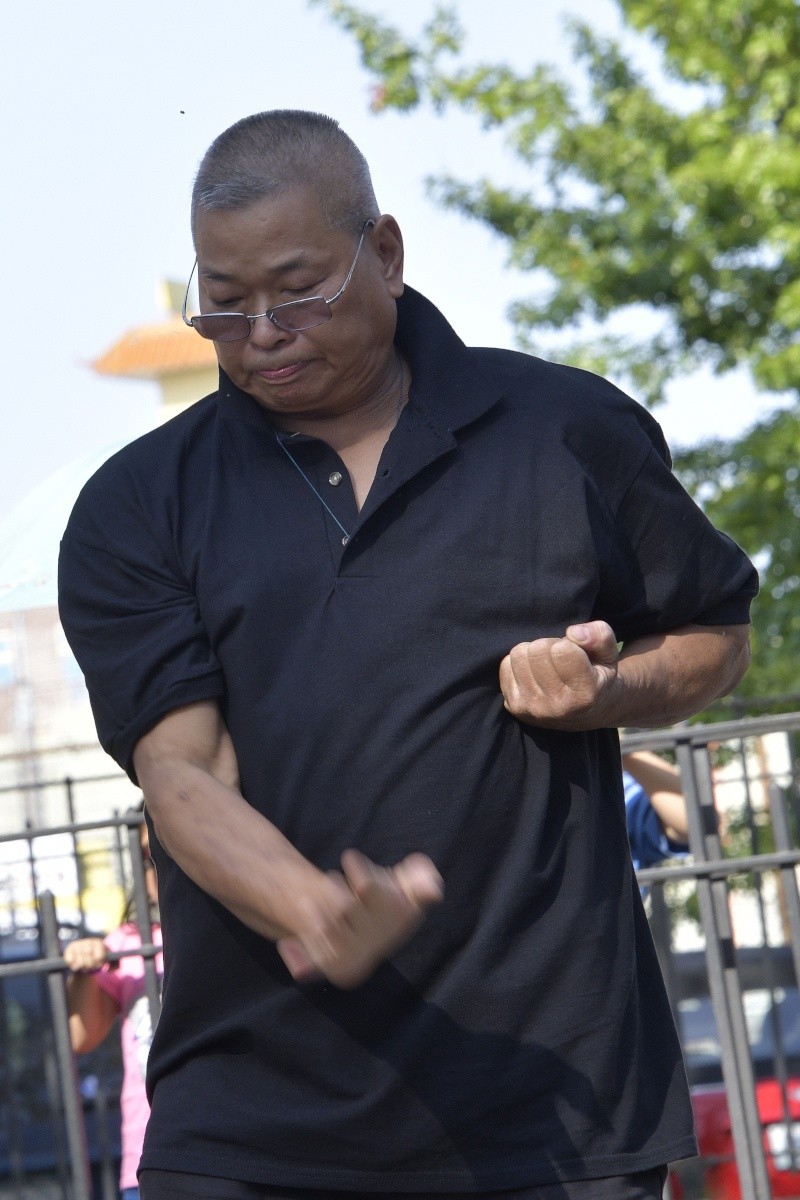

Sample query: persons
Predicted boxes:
[619,743,691,871]
[66,804,163,1200]
[57,112,759,1200]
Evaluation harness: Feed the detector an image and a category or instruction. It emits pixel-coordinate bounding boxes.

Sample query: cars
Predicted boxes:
[0,920,124,1188]
[648,936,800,1200]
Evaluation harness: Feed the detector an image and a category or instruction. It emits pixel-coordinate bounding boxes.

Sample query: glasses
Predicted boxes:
[182,220,375,342]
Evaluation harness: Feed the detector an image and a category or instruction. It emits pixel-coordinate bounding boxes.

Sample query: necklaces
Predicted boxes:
[271,349,404,541]
